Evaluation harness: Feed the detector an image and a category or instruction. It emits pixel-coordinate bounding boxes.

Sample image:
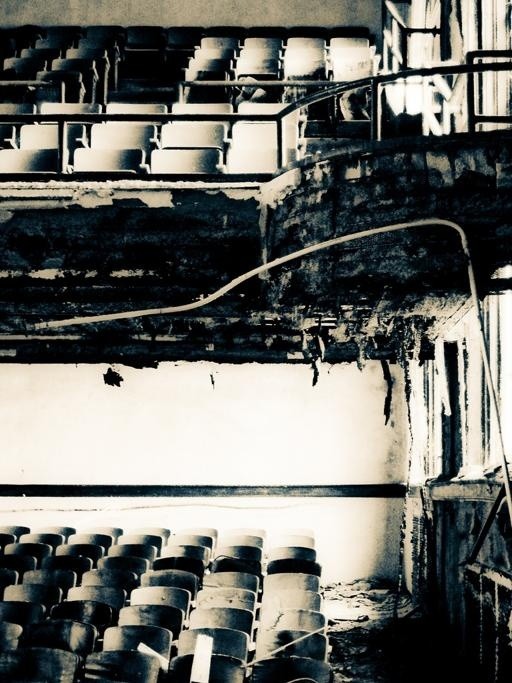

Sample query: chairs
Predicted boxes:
[0,23,374,175]
[0,522,329,682]
